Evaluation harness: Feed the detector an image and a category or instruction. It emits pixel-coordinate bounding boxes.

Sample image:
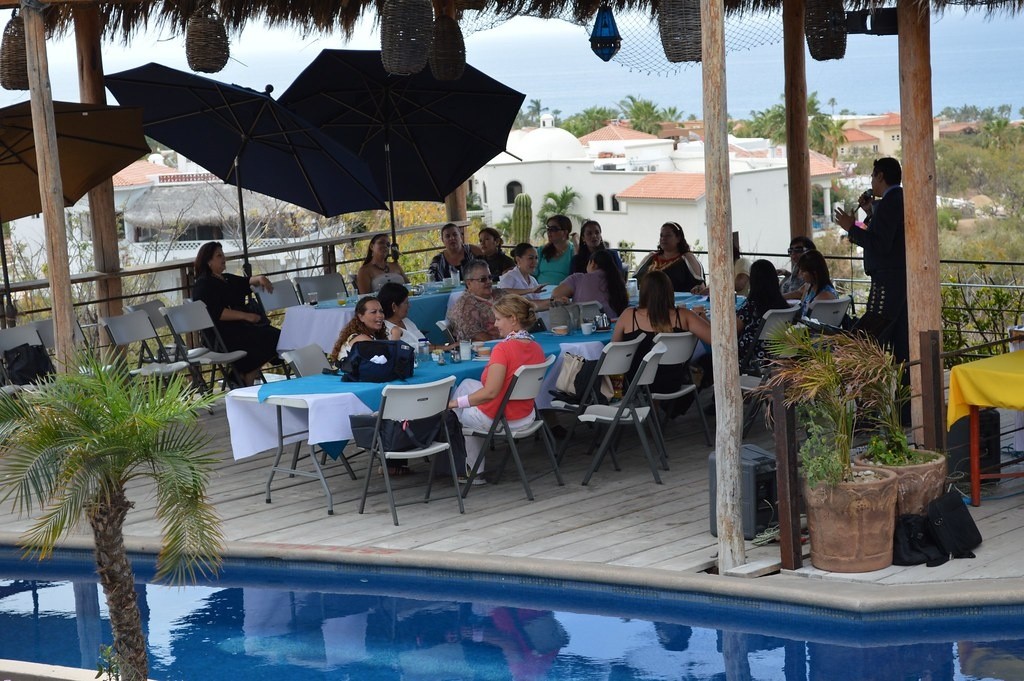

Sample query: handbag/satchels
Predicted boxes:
[556,351,614,403]
[322,340,414,382]
[891,488,983,566]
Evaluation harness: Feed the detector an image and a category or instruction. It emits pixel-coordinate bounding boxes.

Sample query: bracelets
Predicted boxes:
[457,395,470,408]
[434,345,436,349]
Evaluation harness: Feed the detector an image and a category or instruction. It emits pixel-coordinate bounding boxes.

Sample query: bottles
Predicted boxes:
[418,338,430,362]
[610,373,623,397]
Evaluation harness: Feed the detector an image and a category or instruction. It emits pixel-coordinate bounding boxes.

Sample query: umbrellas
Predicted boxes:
[0,99,152,328]
[103,63,391,300]
[276,50,526,259]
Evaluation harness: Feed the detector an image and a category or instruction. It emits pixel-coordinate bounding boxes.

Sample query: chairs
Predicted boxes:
[1,299,247,418]
[359,374,464,526]
[250,279,304,379]
[652,330,714,458]
[808,296,859,333]
[462,355,564,503]
[281,343,366,479]
[294,273,348,305]
[578,343,670,486]
[552,333,648,475]
[737,302,806,441]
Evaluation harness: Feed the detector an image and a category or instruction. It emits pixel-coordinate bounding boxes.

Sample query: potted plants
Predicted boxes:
[833,335,946,514]
[760,321,896,573]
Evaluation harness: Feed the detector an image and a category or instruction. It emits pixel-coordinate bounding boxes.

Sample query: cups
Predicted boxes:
[336,292,347,306]
[450,270,460,287]
[581,323,596,335]
[627,278,638,296]
[473,342,484,351]
[307,292,319,306]
[693,304,705,319]
[347,289,358,302]
[478,347,490,355]
[552,326,569,335]
[460,340,472,361]
[443,278,452,287]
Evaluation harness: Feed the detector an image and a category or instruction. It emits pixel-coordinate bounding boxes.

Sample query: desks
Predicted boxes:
[277,281,560,362]
[225,296,748,516]
[947,351,1024,508]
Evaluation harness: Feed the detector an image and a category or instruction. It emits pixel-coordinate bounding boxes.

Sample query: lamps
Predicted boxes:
[589,2,622,62]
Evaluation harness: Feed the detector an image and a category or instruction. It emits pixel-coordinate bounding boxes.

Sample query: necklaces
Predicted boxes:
[369,261,390,274]
[553,248,567,256]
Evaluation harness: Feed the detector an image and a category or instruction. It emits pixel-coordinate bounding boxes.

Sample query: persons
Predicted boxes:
[448,294,546,485]
[376,282,460,354]
[191,242,285,387]
[690,242,750,298]
[695,260,791,414]
[834,157,911,427]
[610,270,711,418]
[357,233,410,294]
[776,236,817,293]
[781,249,839,354]
[331,297,411,476]
[428,214,706,342]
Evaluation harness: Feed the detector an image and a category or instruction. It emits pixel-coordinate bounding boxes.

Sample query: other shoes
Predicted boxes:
[849,413,878,439]
[456,473,488,485]
[535,425,567,441]
[377,466,409,476]
[703,403,718,415]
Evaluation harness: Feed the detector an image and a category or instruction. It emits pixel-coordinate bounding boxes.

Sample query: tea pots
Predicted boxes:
[594,313,611,330]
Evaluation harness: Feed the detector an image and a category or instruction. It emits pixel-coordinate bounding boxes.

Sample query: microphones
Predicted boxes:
[854,189,873,212]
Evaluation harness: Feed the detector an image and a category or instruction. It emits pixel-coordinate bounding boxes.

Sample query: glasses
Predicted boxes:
[468,275,494,283]
[545,224,566,233]
[374,239,391,248]
[787,246,810,254]
